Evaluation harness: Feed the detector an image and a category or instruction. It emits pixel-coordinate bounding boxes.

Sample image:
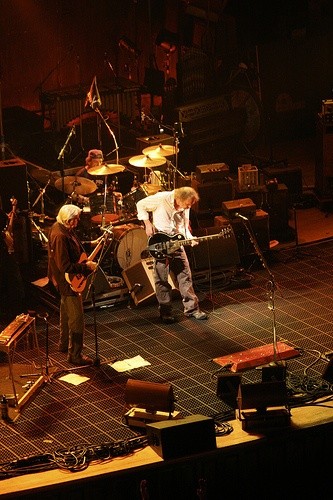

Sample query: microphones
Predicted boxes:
[135,283,141,286]
[234,213,249,220]
[100,227,112,234]
[140,108,145,121]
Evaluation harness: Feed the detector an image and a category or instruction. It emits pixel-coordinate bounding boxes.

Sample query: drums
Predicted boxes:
[103,222,148,273]
[90,213,120,227]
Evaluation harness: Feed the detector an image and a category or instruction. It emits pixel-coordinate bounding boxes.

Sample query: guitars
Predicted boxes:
[146,227,232,260]
[65,225,114,292]
[1,196,18,255]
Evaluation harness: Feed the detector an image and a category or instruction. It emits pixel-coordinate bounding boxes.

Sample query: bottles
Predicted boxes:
[0,396,8,419]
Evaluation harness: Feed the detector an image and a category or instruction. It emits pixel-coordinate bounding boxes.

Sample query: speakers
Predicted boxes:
[144,414,217,460]
[121,257,181,307]
[82,265,110,302]
[124,379,175,411]
[215,365,289,410]
[185,167,303,270]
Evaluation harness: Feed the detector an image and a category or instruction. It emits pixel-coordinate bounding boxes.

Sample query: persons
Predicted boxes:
[75,149,123,201]
[48,204,114,365]
[137,187,206,323]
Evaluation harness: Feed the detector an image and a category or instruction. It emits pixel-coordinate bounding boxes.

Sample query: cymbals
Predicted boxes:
[87,164,126,176]
[142,145,179,157]
[54,176,97,195]
[128,155,167,167]
[30,169,60,187]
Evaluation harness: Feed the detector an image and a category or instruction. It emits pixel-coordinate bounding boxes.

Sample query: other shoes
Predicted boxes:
[81,355,93,365]
[160,315,174,323]
[190,310,207,320]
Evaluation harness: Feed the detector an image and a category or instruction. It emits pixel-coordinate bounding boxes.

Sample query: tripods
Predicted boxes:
[63,233,117,383]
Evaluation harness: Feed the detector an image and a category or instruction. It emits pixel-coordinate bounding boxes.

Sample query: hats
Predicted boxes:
[88,149,103,158]
